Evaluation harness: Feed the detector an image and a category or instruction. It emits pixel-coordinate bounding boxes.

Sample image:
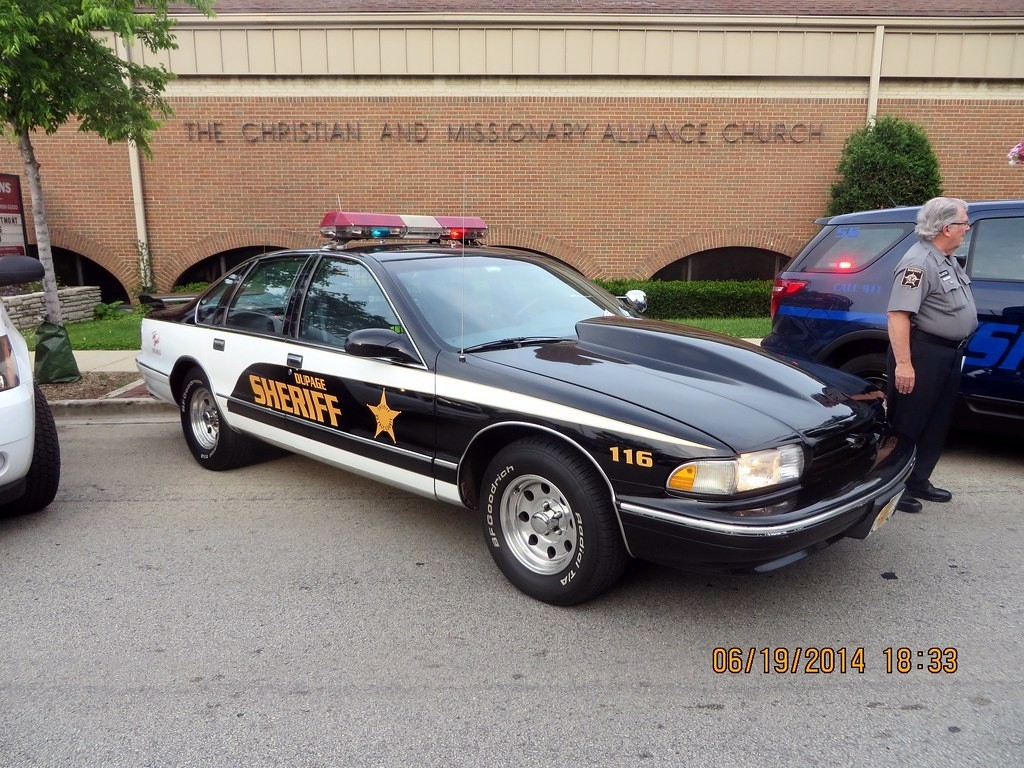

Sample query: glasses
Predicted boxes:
[950,221,971,227]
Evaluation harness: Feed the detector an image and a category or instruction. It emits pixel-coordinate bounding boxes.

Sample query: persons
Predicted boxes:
[886,197,978,513]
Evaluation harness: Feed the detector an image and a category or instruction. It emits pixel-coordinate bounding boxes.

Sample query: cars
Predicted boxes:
[136,213,917,608]
[760,199,1024,426]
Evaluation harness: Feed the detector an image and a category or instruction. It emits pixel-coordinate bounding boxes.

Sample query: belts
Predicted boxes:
[910,328,965,349]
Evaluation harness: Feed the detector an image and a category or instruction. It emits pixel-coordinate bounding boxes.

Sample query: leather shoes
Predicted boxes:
[910,483,952,502]
[895,489,922,512]
